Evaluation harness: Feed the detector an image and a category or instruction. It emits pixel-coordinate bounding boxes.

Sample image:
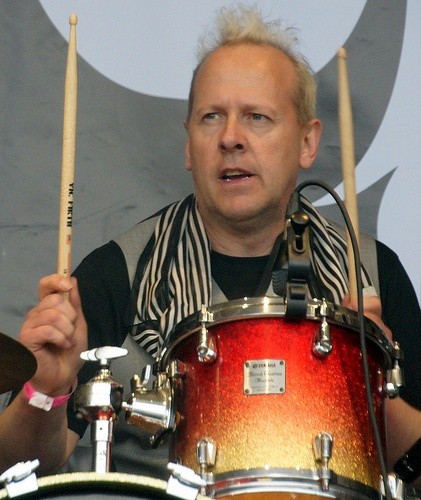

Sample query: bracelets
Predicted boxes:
[20,376,78,410]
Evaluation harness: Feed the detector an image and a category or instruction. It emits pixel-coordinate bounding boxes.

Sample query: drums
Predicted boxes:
[0,459,219,500]
[128,296,401,500]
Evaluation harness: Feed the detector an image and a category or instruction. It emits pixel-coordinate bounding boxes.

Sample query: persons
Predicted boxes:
[1,2,421,500]
